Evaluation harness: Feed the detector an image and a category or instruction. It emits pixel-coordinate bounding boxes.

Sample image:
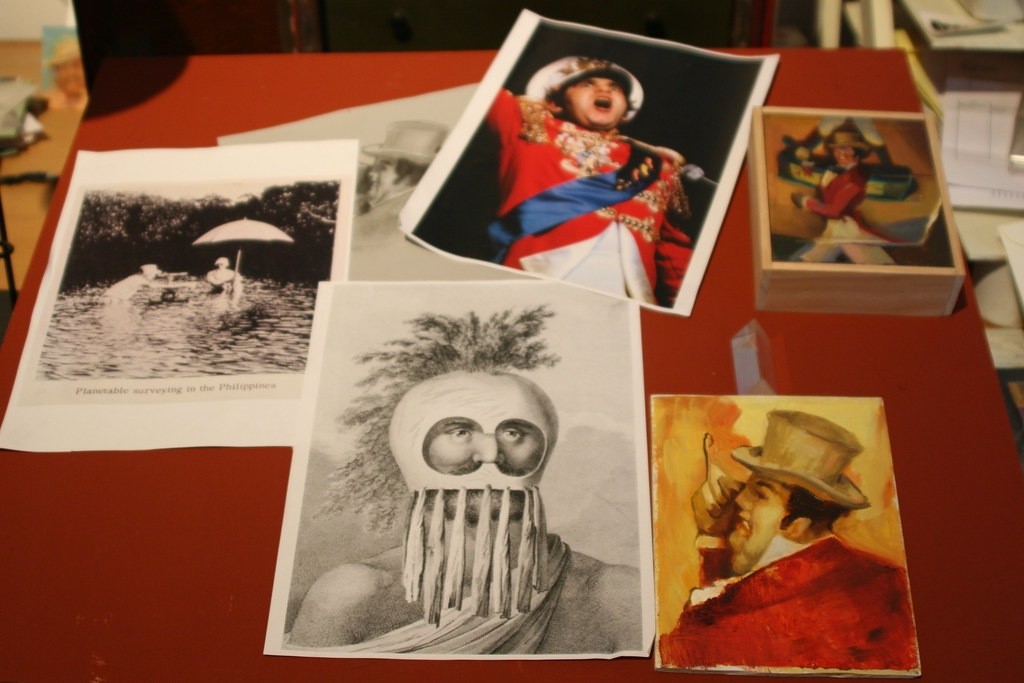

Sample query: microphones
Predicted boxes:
[681,163,719,188]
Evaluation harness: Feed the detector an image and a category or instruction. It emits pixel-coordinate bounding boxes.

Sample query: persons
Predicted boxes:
[486,56,694,304]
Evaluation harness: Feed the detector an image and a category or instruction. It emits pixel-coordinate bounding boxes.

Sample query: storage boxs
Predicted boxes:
[748,106,966,319]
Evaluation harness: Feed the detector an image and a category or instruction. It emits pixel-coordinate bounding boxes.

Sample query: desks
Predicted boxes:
[0,49,1024,683]
